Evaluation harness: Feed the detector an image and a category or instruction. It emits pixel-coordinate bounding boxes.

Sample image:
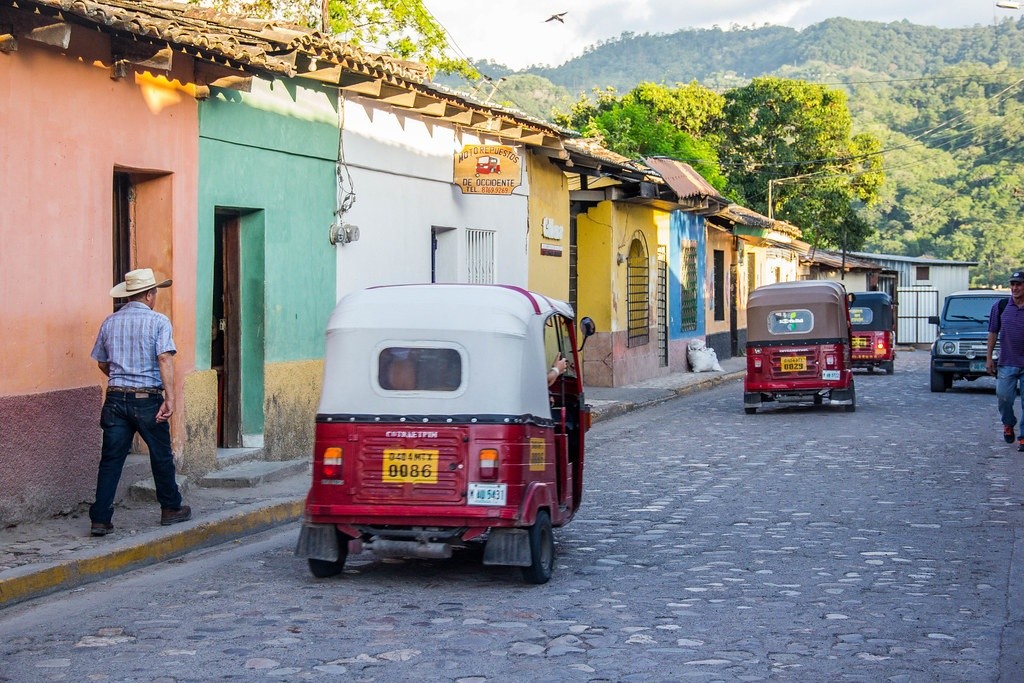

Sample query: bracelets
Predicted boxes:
[212,367,218,372]
[550,367,560,377]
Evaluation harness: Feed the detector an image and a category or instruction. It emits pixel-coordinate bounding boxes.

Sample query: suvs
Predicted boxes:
[926,290,1013,390]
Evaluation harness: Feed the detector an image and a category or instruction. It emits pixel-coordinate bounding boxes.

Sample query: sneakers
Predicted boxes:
[1016,440,1024,451]
[1003,425,1015,443]
[161,506,191,525]
[91,522,113,535]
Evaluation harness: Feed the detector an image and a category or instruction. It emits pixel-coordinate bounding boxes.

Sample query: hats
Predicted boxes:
[109,268,173,297]
[1008,272,1024,282]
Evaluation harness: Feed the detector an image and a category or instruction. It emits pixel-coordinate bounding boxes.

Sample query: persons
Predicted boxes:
[985,272,1024,452]
[211,315,224,373]
[88,268,192,535]
[547,352,567,387]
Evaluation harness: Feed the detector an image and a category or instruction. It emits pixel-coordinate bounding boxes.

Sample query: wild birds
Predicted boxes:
[544,11,568,23]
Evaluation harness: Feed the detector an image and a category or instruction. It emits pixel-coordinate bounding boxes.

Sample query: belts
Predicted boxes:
[107,387,163,394]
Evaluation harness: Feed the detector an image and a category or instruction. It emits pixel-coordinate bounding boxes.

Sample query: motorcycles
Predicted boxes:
[847,292,900,375]
[293,282,596,580]
[743,278,857,413]
